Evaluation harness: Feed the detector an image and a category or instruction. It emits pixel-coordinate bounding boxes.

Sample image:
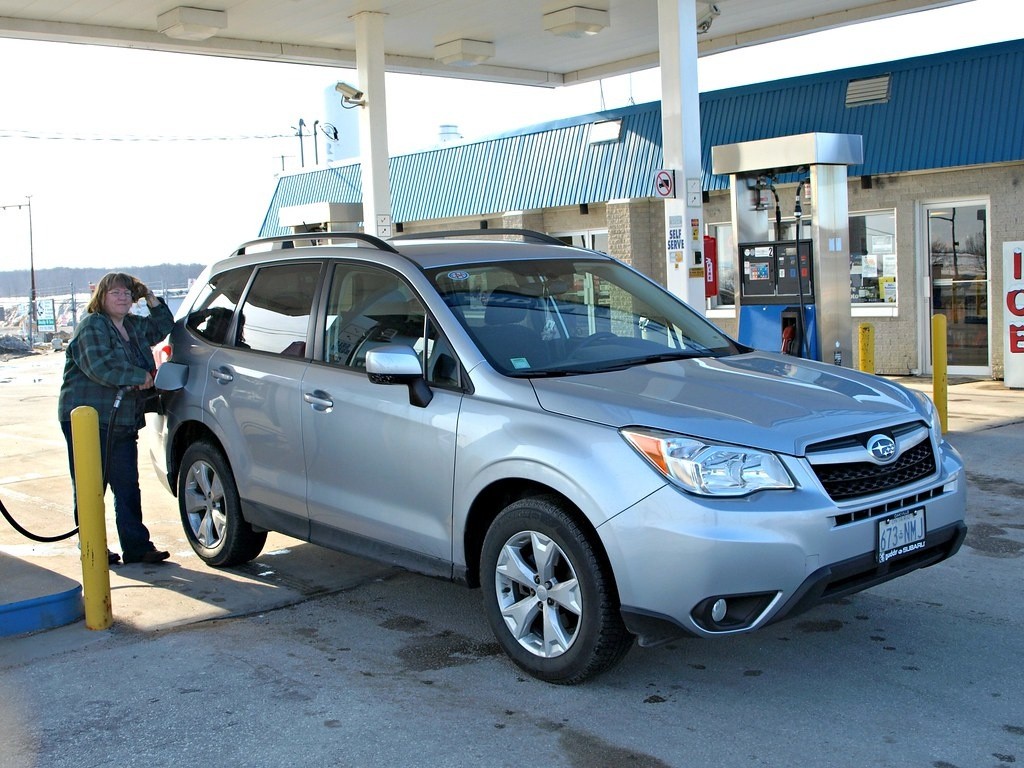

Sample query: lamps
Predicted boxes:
[434,38,495,67]
[543,6,610,38]
[156,7,227,41]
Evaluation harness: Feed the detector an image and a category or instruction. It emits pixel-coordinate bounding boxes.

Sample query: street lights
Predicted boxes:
[0,197,39,320]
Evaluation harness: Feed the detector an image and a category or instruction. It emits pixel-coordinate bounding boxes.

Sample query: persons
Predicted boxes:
[56,273,176,566]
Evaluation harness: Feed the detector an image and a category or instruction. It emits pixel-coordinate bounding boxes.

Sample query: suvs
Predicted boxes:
[148,225,967,687]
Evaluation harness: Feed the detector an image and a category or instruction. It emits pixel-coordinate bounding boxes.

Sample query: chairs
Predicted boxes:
[338,290,414,370]
[474,284,548,372]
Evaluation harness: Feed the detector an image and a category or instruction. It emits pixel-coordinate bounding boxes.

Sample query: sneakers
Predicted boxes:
[122,541,170,564]
[108,549,120,563]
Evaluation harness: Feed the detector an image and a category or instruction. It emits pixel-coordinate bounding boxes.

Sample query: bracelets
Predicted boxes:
[145,290,154,300]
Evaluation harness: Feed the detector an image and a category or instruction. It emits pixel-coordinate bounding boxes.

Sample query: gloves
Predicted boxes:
[131,282,147,302]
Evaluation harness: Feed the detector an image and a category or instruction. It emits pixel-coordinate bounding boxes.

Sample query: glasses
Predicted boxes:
[106,290,132,297]
